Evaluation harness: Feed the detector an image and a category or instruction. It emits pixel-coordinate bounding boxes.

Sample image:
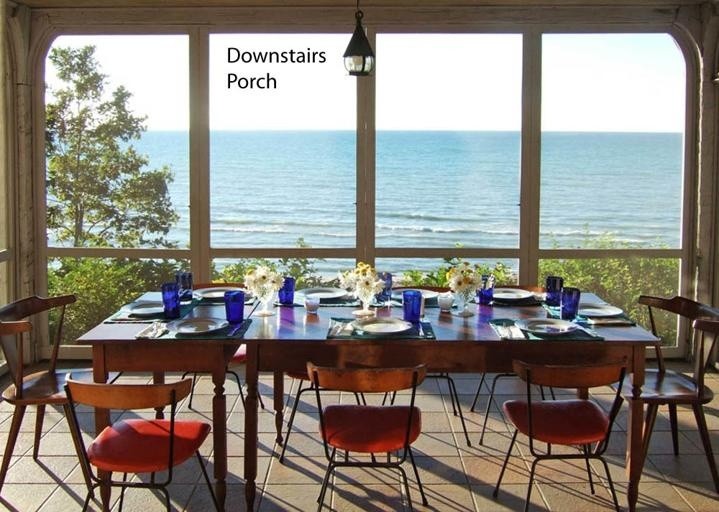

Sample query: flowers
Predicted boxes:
[337,262,387,300]
[445,265,483,300]
[244,265,285,298]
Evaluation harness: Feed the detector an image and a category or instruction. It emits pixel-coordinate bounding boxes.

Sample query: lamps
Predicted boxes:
[342,10,375,76]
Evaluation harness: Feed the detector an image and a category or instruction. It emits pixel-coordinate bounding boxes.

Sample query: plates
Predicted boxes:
[490,287,533,300]
[350,318,414,334]
[173,318,229,335]
[301,287,348,300]
[193,287,248,299]
[577,302,623,317]
[391,288,439,300]
[118,301,164,317]
[514,316,580,336]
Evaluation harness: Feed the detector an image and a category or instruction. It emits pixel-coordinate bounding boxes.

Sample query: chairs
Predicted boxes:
[0,283,719,512]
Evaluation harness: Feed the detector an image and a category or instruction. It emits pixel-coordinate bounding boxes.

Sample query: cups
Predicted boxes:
[174,270,193,301]
[475,305,494,337]
[303,313,320,333]
[276,306,295,336]
[545,275,564,306]
[402,290,422,321]
[350,309,375,323]
[561,286,580,321]
[223,290,244,323]
[302,296,320,314]
[375,271,392,301]
[477,274,493,305]
[161,282,181,320]
[435,294,455,313]
[278,277,295,305]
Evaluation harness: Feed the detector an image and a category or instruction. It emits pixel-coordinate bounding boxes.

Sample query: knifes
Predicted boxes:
[576,324,595,338]
